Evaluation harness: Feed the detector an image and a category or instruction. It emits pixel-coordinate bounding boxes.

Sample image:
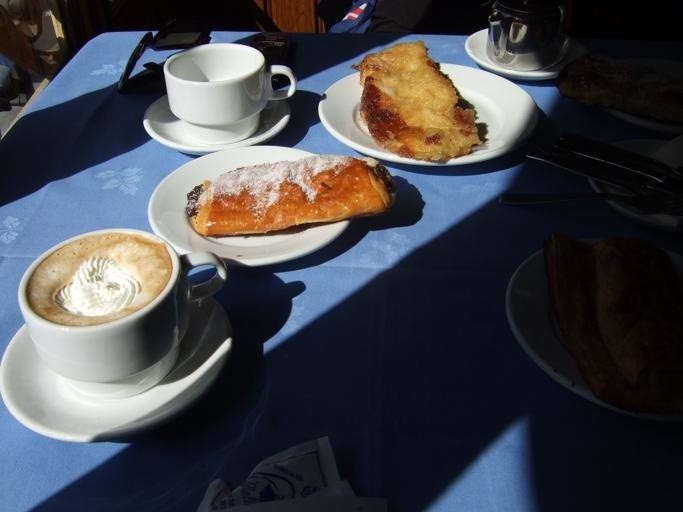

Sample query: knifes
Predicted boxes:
[517,122,683,208]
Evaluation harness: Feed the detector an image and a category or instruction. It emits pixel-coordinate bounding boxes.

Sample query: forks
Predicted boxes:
[494,188,683,219]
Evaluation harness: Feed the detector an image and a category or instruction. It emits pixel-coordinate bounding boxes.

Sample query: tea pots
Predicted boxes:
[482,0,573,72]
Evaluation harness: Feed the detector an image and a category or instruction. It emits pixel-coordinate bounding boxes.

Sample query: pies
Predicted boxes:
[559,64,682,125]
[544,233,682,414]
[352,41,485,164]
[186,155,398,239]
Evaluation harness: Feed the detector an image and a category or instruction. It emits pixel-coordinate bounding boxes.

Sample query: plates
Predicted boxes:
[149,147,354,266]
[464,27,578,83]
[506,243,680,421]
[143,93,292,157]
[0,298,232,442]
[591,136,680,228]
[316,63,539,168]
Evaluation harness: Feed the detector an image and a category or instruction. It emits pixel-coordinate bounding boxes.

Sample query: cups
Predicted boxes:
[17,228,228,400]
[162,42,298,144]
